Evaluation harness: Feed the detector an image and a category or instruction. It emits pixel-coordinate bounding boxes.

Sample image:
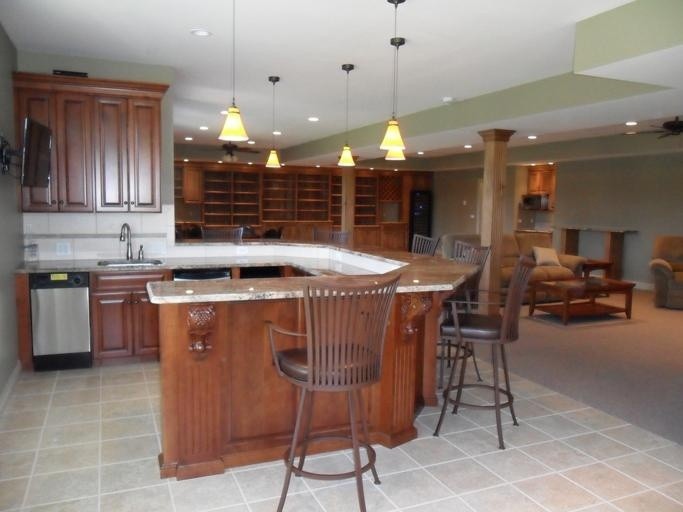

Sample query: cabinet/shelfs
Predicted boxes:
[525,163,557,211]
[9,71,95,213]
[93,75,170,215]
[173,158,432,251]
[87,269,166,364]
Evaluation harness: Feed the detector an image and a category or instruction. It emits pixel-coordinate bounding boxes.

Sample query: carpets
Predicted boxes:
[439,286,683,447]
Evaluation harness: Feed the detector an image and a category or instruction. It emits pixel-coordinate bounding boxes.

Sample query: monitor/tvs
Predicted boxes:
[20,117,52,188]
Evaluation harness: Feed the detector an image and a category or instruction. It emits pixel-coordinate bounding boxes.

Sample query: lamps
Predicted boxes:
[214,1,412,170]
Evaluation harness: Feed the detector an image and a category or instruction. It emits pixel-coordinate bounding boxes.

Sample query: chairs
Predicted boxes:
[646,233,682,311]
[264,273,404,512]
[410,232,440,257]
[430,255,537,450]
[435,240,493,389]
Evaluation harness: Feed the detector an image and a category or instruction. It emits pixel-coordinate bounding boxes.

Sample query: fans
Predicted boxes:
[618,116,683,142]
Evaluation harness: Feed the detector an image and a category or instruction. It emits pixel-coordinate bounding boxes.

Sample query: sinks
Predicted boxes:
[97,260,165,266]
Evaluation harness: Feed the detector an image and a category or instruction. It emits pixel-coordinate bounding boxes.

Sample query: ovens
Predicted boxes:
[27,273,94,372]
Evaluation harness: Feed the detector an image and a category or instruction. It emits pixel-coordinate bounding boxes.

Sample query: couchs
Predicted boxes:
[439,227,587,308]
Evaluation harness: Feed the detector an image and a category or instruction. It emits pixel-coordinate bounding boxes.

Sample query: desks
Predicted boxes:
[578,255,613,300]
[554,222,638,282]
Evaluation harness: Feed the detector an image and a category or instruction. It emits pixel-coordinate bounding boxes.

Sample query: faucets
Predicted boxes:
[119,222,135,259]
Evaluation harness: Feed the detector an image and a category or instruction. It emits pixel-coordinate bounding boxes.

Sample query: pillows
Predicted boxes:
[529,244,561,267]
